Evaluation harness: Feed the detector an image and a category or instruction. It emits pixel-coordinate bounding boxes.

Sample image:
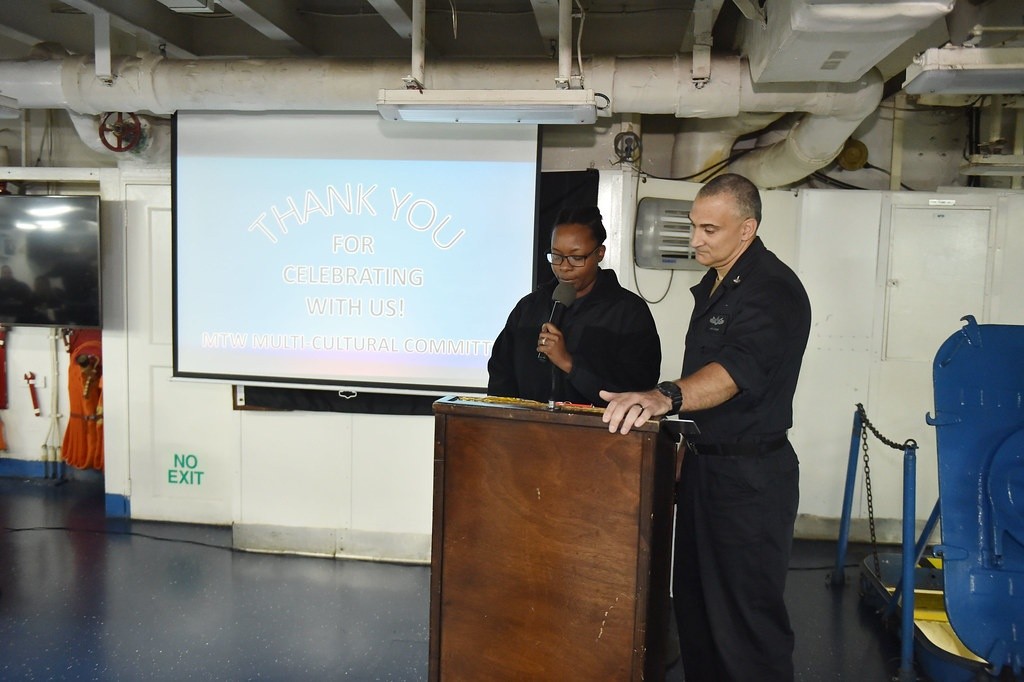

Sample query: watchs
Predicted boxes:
[655,381,683,418]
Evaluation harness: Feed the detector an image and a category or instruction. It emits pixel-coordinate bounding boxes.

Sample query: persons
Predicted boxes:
[597,170,813,682]
[485,204,663,410]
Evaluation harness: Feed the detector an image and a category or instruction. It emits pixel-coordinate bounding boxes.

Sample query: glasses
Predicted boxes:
[544,245,601,267]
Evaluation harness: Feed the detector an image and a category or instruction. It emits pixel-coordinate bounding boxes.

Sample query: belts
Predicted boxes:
[686,435,788,456]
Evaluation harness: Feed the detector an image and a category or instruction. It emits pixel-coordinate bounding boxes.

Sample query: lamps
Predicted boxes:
[377,88,598,126]
[902,47,1024,95]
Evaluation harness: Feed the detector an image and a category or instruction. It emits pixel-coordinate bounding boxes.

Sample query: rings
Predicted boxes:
[541,338,547,345]
[634,402,644,410]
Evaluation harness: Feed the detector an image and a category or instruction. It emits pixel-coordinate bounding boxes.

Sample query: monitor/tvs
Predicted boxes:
[0,194,102,329]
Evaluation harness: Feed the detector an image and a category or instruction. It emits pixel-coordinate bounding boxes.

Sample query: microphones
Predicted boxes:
[538,283,578,362]
